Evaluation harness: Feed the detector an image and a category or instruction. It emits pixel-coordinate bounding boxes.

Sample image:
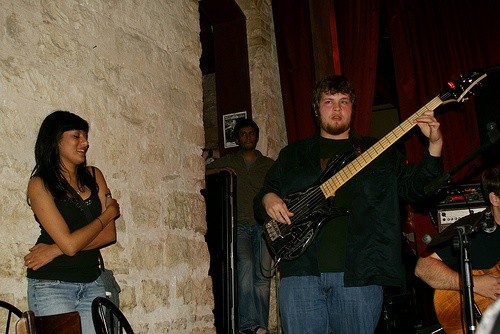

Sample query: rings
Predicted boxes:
[105,193,112,197]
[27,260,31,263]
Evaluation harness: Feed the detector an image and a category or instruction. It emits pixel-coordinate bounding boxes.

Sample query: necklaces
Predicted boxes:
[65,178,83,195]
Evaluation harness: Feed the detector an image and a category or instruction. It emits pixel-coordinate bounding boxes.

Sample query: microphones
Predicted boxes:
[423,140,493,196]
[440,218,494,238]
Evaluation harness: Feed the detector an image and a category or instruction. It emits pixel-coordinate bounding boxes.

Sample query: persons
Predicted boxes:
[256,76,443,334]
[23,110,121,334]
[202,118,277,334]
[413,164,500,334]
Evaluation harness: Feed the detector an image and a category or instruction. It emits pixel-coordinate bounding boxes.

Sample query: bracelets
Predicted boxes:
[96,218,104,231]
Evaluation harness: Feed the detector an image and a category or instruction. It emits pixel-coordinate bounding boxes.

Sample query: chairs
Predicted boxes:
[0,300,23,334]
[15,311,82,334]
[91,297,135,334]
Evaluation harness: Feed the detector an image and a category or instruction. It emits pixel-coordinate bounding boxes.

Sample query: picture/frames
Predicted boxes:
[222,111,248,149]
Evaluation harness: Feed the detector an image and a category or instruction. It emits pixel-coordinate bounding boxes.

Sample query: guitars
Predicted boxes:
[263,71,488,262]
[433,260,500,334]
